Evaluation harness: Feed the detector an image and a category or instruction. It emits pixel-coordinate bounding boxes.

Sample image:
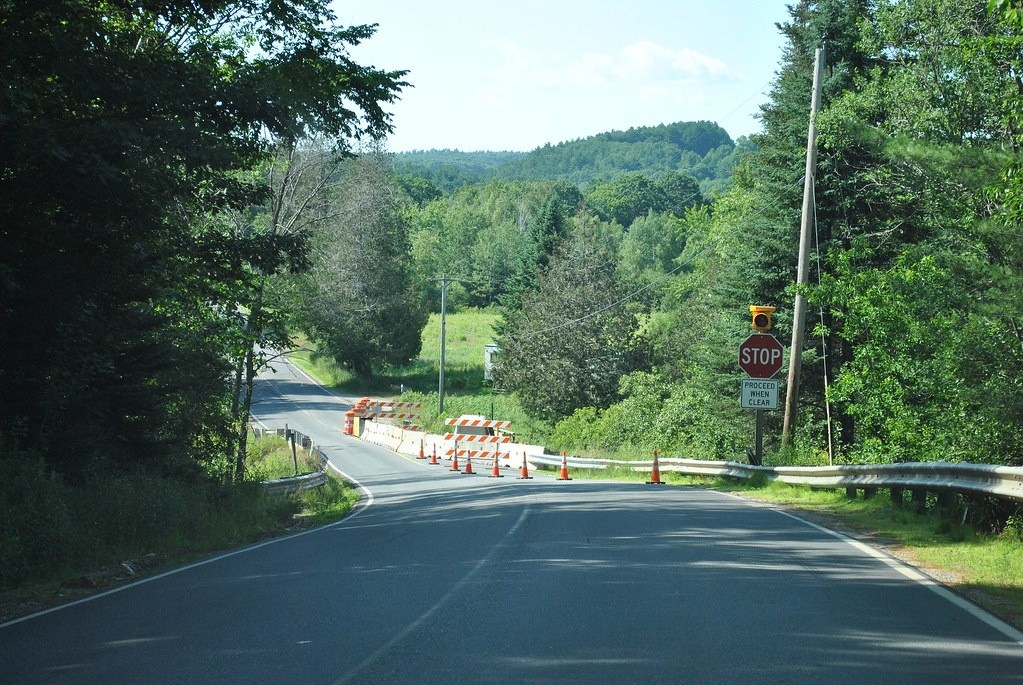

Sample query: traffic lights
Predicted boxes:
[753,313,772,332]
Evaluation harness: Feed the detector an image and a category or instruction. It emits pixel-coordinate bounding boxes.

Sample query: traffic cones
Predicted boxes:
[447,440,463,471]
[461,449,478,475]
[516,451,534,480]
[487,452,504,478]
[643,450,667,485]
[555,449,572,481]
[416,439,427,459]
[428,442,439,465]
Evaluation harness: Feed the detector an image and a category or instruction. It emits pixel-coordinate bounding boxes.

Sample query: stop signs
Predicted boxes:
[736,332,784,380]
